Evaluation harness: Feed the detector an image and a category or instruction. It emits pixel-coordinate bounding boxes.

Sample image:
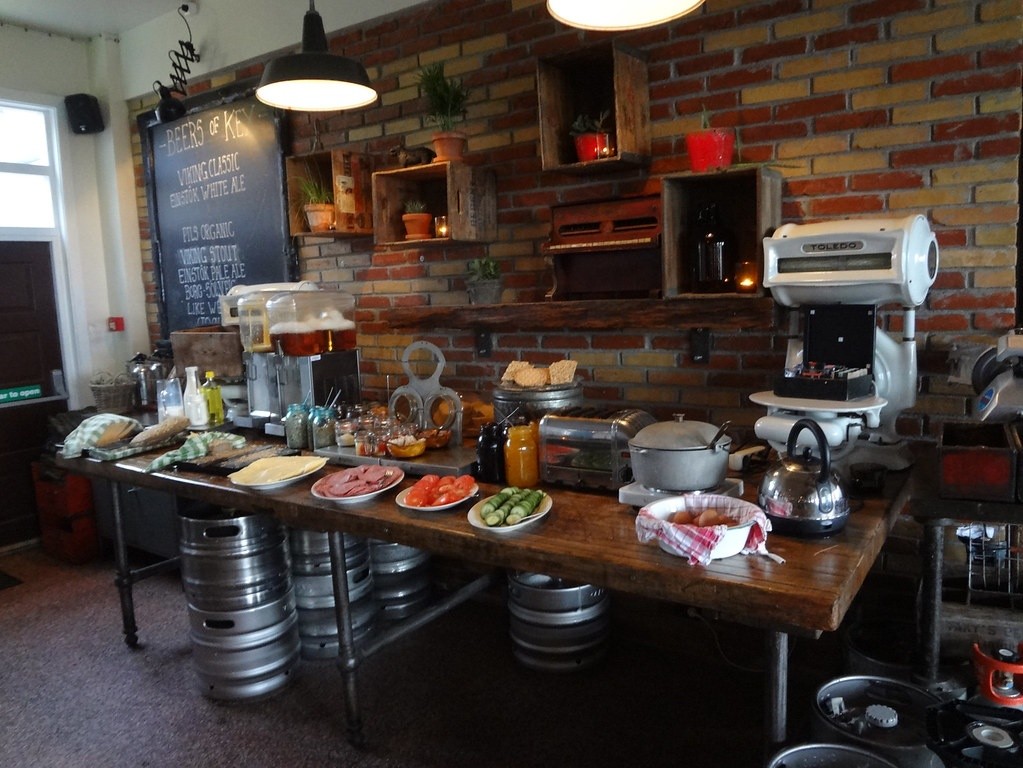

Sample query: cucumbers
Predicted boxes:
[480,486,547,528]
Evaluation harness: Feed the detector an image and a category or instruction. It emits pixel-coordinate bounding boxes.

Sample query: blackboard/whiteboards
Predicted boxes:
[137,73,300,358]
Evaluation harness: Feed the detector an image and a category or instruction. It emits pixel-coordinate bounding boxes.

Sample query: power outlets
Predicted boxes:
[184,2,196,15]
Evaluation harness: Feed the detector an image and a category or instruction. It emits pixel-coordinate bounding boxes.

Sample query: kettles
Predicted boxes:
[127,347,174,408]
[758,418,850,537]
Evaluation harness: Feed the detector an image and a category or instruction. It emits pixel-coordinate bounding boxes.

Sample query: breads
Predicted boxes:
[130,416,190,447]
[501,359,578,386]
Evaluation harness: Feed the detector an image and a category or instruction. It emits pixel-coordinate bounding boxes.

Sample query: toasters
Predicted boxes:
[538,403,657,493]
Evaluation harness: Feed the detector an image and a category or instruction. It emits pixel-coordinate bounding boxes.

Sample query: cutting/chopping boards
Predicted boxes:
[172,445,301,476]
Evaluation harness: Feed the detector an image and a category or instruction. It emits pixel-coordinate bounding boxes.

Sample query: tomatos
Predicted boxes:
[406,475,475,507]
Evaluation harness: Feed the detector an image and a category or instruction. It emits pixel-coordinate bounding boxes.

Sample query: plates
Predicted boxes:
[395,481,478,511]
[231,457,326,489]
[468,490,553,533]
[312,466,405,503]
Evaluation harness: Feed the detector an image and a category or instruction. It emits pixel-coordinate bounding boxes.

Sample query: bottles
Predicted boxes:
[287,401,418,457]
[476,417,539,487]
[156,366,224,426]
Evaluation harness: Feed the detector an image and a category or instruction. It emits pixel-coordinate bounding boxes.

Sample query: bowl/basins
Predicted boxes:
[645,497,756,559]
[384,439,426,458]
[416,430,452,449]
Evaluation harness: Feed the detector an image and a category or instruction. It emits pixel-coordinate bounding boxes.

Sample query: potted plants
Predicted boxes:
[413,61,470,163]
[464,256,504,305]
[401,198,433,239]
[285,155,337,233]
[569,107,615,162]
[686,103,734,172]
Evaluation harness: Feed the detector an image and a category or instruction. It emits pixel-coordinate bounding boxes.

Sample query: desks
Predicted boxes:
[55,437,914,768]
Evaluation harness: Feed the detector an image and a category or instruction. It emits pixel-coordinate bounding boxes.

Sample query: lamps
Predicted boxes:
[545,0,706,32]
[146,5,200,129]
[254,0,378,113]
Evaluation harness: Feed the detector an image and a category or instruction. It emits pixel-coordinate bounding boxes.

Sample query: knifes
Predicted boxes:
[196,443,273,467]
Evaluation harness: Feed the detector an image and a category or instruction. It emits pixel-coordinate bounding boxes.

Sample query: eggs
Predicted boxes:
[671,509,739,527]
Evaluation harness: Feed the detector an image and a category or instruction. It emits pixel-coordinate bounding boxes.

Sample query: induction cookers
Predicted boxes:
[620,478,744,512]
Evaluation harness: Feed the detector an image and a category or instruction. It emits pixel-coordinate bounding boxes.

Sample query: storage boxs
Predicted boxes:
[170,290,357,383]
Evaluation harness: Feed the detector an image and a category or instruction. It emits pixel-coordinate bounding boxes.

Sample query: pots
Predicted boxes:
[490,375,585,424]
[628,412,733,491]
